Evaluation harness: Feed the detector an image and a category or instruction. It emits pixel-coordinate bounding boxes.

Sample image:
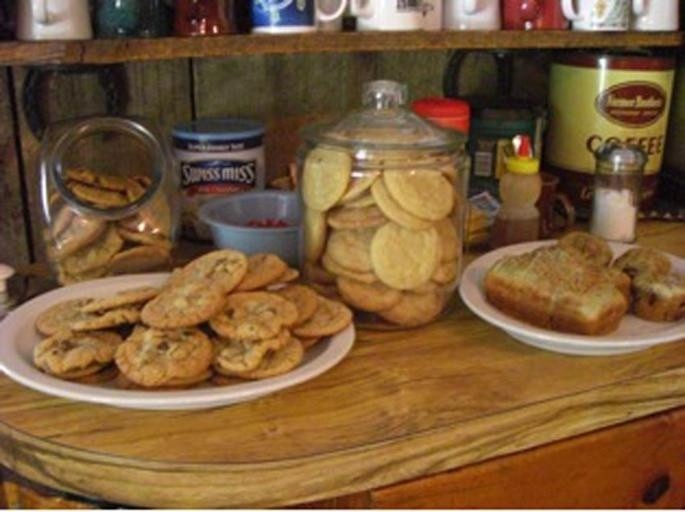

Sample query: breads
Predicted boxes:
[482,230,684,336]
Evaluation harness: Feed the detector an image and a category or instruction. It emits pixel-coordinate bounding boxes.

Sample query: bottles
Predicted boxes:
[491,134,544,248]
[588,140,652,244]
[294,79,473,333]
[34,113,184,289]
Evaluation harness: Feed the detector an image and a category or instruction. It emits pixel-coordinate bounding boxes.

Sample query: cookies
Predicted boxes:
[301,144,461,327]
[40,163,173,286]
[32,248,354,388]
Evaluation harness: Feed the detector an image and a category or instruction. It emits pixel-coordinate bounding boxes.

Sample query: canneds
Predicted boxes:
[167,118,267,241]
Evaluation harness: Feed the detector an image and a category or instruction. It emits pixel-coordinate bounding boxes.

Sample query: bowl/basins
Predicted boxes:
[195,189,305,267]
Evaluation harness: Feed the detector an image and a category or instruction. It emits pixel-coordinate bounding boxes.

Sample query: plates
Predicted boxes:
[456,238,685,360]
[0,269,357,412]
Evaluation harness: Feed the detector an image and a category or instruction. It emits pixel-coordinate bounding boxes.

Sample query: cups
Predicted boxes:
[12,0,681,44]
[536,172,577,240]
